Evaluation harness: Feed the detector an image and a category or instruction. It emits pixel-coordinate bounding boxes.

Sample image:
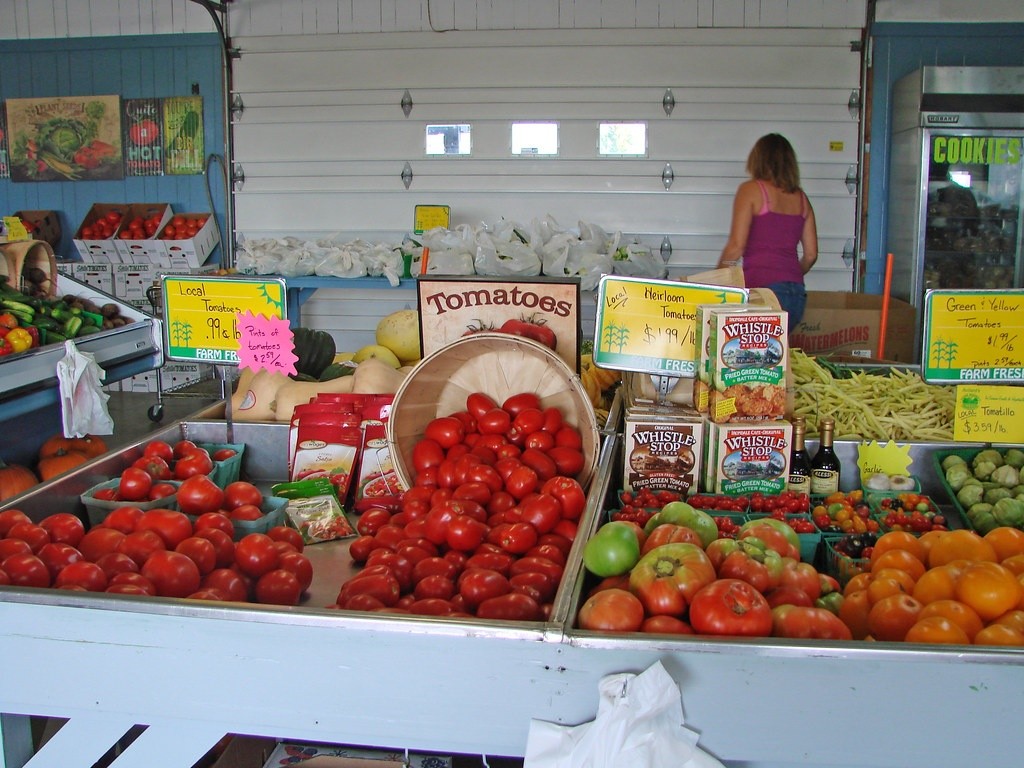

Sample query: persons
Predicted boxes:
[716,133,818,333]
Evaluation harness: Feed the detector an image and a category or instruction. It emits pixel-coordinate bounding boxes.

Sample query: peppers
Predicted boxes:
[0,312,40,356]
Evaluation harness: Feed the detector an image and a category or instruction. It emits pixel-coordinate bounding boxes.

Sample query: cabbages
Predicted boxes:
[33,118,92,162]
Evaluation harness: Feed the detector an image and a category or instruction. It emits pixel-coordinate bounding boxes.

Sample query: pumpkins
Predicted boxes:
[0,430,110,502]
[222,308,623,430]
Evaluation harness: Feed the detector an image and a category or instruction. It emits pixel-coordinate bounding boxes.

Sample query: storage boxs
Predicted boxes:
[803,438,989,507]
[749,508,813,518]
[12,211,62,248]
[685,497,750,514]
[809,492,875,512]
[197,442,246,488]
[706,514,747,526]
[615,490,684,510]
[932,448,1024,530]
[859,475,921,499]
[57,202,222,393]
[748,514,822,564]
[159,463,218,521]
[693,303,774,412]
[187,495,291,540]
[608,510,661,522]
[824,537,870,589]
[804,364,1024,517]
[702,417,715,493]
[630,397,693,409]
[713,423,793,495]
[710,310,788,423]
[871,494,942,514]
[82,477,181,528]
[875,514,955,537]
[809,513,885,536]
[788,292,917,362]
[619,416,703,496]
[625,408,702,418]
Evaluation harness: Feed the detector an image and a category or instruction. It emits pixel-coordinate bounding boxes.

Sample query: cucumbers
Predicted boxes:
[0,273,102,339]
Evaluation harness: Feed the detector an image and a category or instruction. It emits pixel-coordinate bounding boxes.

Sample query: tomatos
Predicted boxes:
[129,120,159,145]
[0,440,315,607]
[571,489,1024,649]
[499,310,556,351]
[78,210,209,250]
[460,319,501,335]
[325,392,586,622]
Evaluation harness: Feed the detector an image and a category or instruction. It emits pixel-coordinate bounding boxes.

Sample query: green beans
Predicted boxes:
[789,348,959,441]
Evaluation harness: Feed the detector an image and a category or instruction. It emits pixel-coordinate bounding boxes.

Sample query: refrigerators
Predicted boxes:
[888,65,1024,365]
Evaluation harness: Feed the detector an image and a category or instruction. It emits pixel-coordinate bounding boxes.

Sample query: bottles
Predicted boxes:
[811,419,841,501]
[788,415,810,496]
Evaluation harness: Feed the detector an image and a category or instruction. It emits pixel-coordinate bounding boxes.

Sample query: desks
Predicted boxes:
[253,268,669,331]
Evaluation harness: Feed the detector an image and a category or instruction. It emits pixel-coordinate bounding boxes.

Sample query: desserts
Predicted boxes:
[693,381,787,425]
